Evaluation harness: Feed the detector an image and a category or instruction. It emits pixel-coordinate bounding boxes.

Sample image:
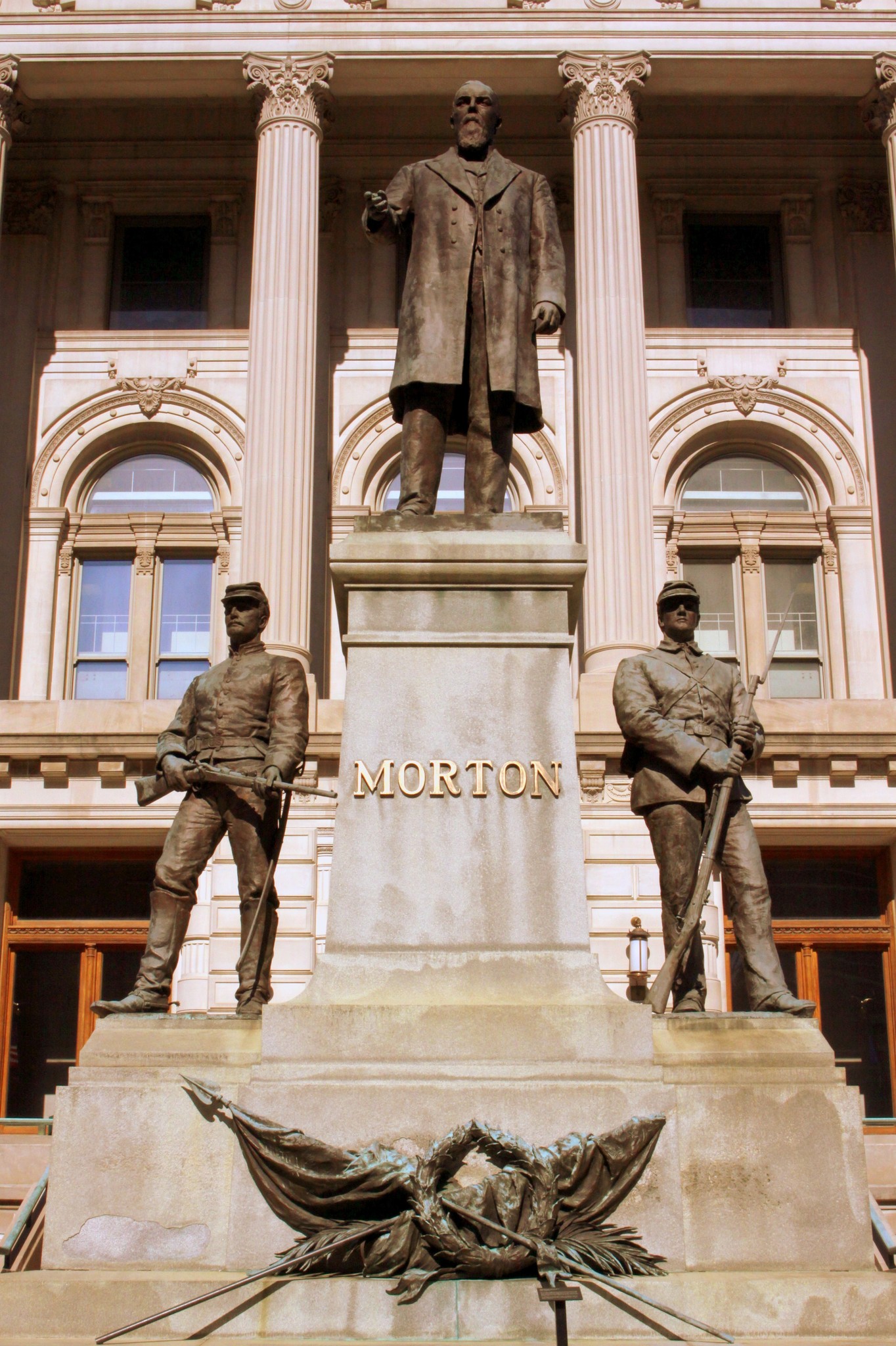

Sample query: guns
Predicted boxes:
[641,589,797,1016]
[133,759,339,807]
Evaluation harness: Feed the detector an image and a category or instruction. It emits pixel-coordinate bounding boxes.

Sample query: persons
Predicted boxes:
[88,582,308,1011]
[361,80,567,513]
[612,579,817,1013]
